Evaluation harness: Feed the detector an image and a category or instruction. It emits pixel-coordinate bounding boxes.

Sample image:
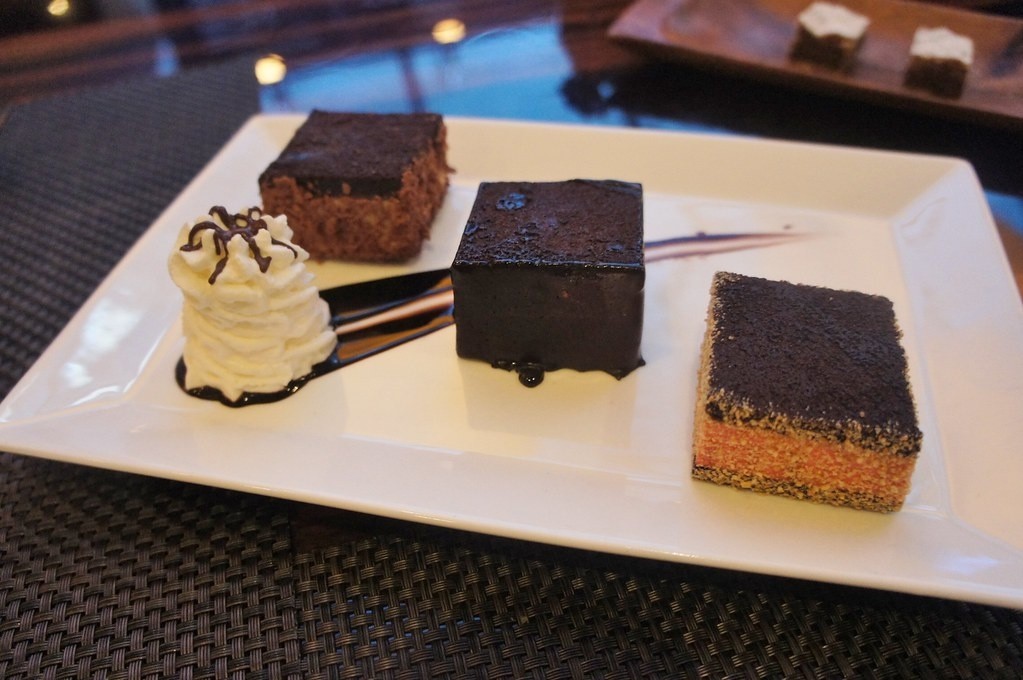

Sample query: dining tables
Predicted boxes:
[1,0,1023,680]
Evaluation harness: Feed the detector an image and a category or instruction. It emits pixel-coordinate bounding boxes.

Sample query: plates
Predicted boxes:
[607,1,1023,128]
[0,111,1023,622]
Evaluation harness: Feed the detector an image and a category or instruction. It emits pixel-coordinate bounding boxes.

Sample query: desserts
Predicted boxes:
[166,107,924,516]
[787,0,975,94]
